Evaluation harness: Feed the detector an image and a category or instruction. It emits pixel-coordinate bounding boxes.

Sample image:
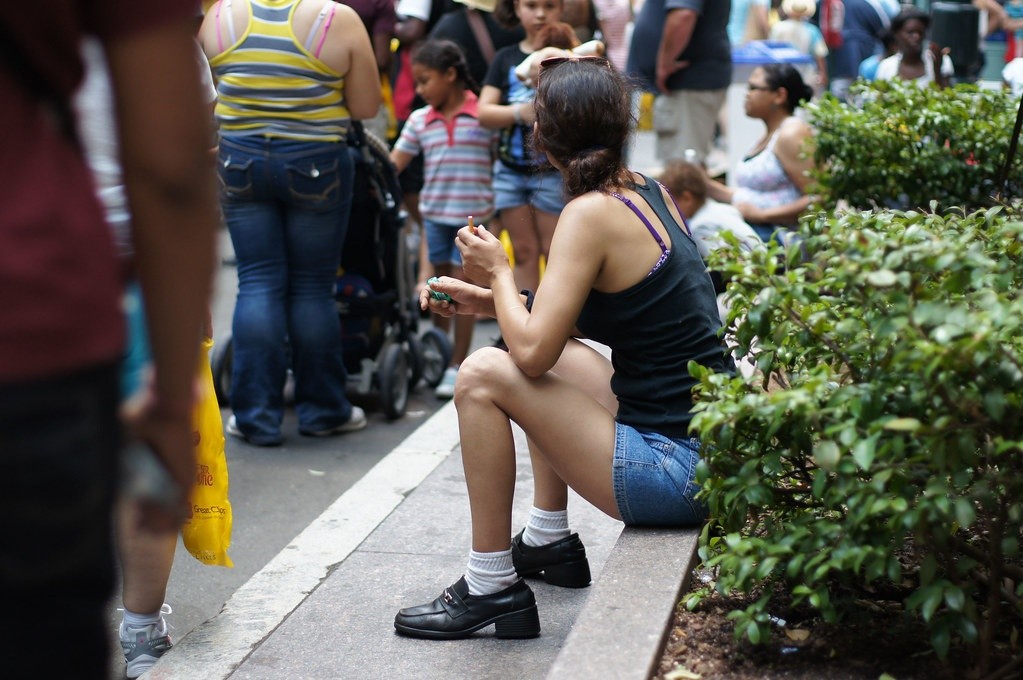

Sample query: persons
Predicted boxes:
[0,2,219,678]
[393,53,742,642]
[76,2,1023,445]
[72,22,219,680]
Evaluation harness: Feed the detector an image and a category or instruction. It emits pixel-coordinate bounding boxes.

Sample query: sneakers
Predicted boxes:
[116,606,172,679]
[224,410,239,434]
[307,404,365,435]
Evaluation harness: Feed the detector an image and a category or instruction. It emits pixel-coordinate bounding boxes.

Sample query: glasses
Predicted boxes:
[536,58,613,112]
[749,84,771,91]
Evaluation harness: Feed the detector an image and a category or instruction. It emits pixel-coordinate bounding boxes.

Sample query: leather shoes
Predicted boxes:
[511,525,592,589]
[395,574,541,641]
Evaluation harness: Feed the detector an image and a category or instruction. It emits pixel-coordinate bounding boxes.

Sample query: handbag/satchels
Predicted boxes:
[180,334,233,570]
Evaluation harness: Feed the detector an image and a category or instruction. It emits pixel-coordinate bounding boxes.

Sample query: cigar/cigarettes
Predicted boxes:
[468,215,473,235]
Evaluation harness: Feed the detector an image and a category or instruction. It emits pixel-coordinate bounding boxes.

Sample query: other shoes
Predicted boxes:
[434,366,458,395]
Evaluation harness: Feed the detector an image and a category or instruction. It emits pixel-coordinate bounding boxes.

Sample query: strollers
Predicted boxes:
[211,131,451,424]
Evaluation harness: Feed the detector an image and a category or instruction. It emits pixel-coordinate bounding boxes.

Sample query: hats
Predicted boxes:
[782,1,816,21]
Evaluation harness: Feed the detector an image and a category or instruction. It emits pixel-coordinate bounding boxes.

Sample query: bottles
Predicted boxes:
[683,148,699,169]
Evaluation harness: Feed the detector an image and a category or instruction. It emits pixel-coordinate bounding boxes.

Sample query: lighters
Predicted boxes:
[426,277,452,306]
